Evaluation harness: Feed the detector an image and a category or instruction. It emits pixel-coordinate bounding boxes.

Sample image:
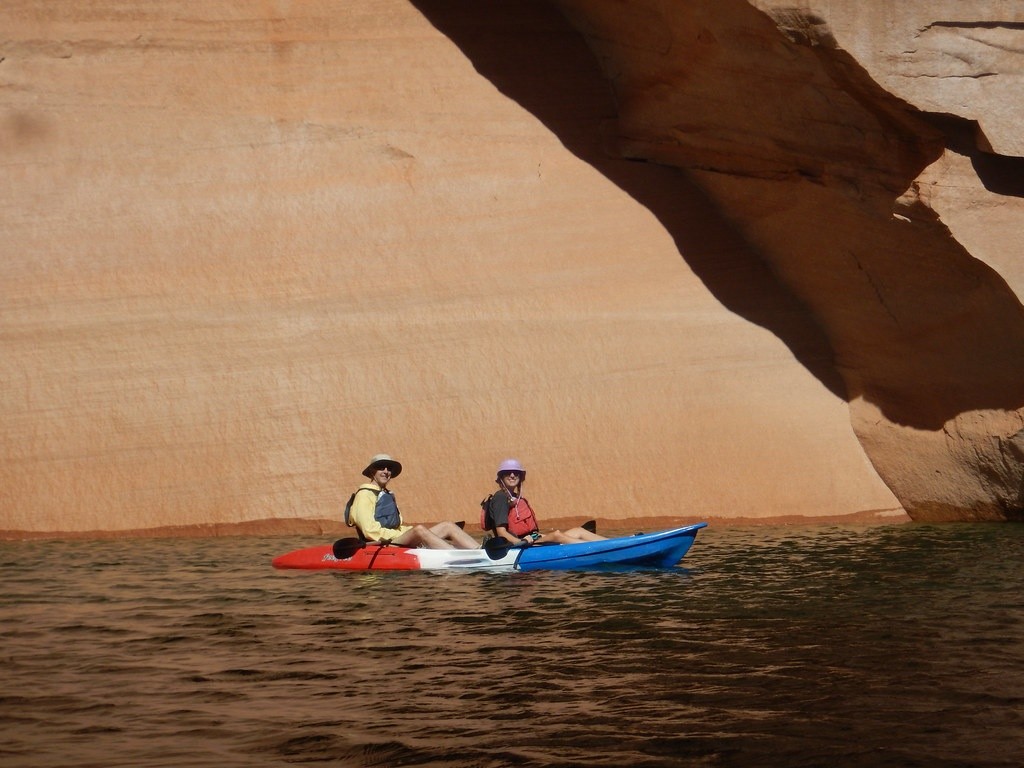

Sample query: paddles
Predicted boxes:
[332,519,468,560]
[483,519,599,561]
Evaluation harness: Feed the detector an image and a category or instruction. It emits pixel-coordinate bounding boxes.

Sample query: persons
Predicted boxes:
[345,454,482,549]
[488,459,609,546]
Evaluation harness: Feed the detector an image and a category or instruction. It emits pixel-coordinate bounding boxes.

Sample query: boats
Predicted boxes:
[272,522,708,575]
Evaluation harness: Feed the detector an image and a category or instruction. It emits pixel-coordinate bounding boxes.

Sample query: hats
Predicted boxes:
[495,459,526,485]
[362,454,402,478]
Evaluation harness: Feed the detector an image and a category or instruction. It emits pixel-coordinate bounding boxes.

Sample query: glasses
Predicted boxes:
[502,470,520,477]
[372,464,393,471]
